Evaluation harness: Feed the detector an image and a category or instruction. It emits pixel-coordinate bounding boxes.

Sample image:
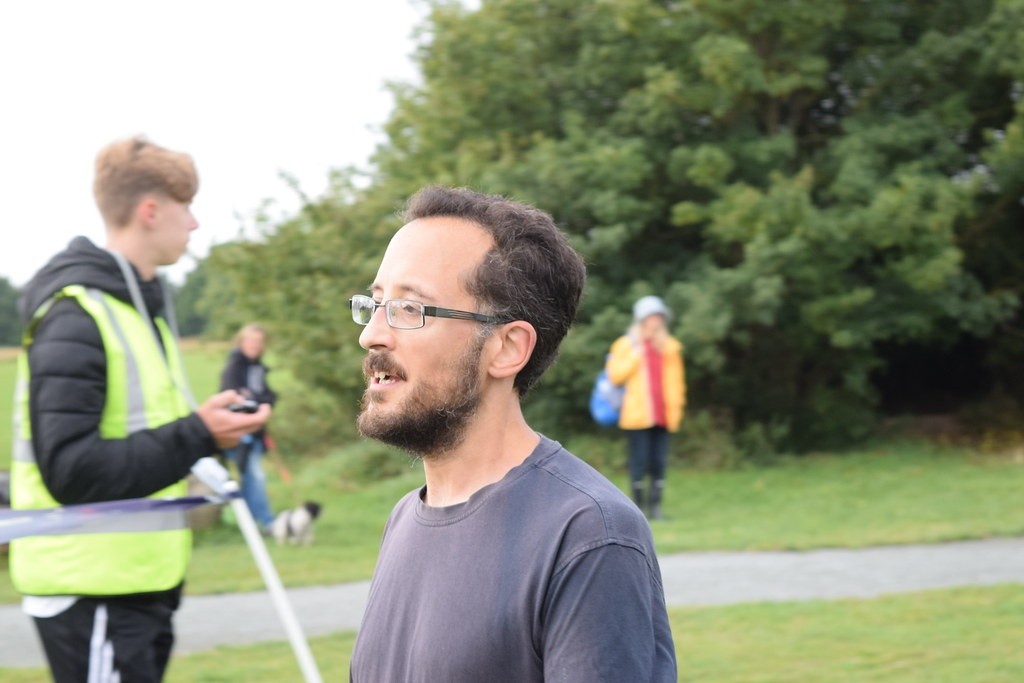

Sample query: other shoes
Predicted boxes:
[651,505,665,520]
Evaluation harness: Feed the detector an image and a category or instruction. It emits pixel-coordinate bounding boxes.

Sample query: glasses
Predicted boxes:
[348,293,515,329]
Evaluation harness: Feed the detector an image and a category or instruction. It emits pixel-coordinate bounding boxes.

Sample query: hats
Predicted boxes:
[632,293,673,321]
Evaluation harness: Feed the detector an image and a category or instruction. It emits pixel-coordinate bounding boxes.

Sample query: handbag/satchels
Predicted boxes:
[588,374,625,427]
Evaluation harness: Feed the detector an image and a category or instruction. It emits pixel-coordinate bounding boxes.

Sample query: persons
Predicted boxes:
[215,322,280,534]
[604,295,686,520]
[5,134,272,683]
[349,184,679,683]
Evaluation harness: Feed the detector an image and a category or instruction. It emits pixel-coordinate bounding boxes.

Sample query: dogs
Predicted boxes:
[262,501,321,546]
